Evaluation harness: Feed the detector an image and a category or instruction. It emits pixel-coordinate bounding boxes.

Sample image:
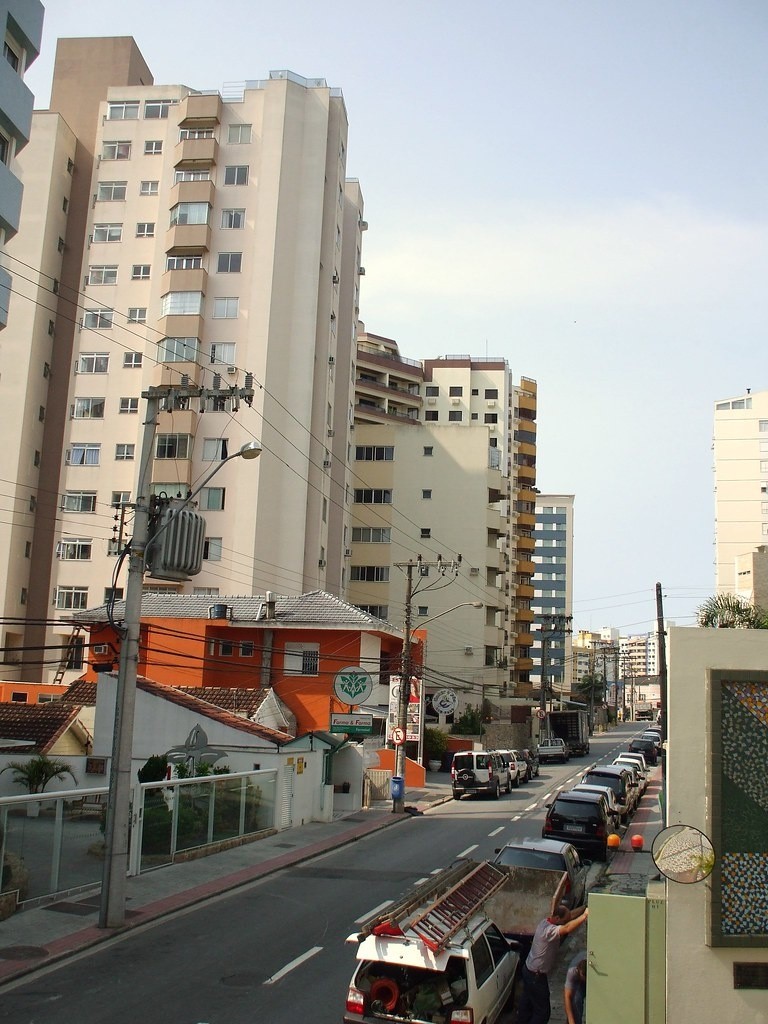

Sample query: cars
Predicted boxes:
[492,838,592,911]
[607,764,645,804]
[612,757,650,795]
[570,783,623,829]
[628,724,662,766]
[541,791,618,861]
[619,752,650,785]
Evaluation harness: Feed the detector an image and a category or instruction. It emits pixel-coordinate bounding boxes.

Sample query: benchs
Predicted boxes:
[70,793,108,820]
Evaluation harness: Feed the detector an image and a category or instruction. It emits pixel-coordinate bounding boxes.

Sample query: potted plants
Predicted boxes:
[424,727,448,773]
[0,752,78,817]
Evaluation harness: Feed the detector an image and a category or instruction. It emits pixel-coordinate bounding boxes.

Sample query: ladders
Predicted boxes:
[409,858,510,959]
[53,626,81,685]
[371,856,473,931]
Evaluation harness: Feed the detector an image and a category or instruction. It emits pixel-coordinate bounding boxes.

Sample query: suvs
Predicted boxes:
[492,750,529,788]
[579,767,639,824]
[451,750,513,800]
[517,749,539,780]
[344,907,524,1024]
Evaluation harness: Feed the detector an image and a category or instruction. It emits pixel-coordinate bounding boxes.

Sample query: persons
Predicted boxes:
[512,903,589,1024]
[562,950,587,1024]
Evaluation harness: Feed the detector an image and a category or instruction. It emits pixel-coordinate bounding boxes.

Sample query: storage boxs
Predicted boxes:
[431,962,467,1024]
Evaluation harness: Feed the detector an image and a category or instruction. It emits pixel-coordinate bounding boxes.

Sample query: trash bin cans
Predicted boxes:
[389,776,404,800]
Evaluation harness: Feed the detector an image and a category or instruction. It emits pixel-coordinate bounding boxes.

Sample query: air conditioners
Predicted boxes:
[323,461,331,468]
[469,568,479,575]
[328,429,336,437]
[345,548,352,555]
[333,275,339,284]
[464,646,473,655]
[319,559,326,567]
[361,221,368,230]
[359,266,365,275]
[350,425,355,430]
[227,366,236,374]
[329,356,334,364]
[356,307,359,314]
[93,645,107,655]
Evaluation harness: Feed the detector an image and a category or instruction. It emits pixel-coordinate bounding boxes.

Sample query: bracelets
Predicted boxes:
[584,903,587,908]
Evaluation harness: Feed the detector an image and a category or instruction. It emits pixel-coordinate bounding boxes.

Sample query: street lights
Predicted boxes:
[97,441,263,931]
[392,601,484,815]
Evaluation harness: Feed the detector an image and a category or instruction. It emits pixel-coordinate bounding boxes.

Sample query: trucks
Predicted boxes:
[634,703,654,722]
[547,711,590,758]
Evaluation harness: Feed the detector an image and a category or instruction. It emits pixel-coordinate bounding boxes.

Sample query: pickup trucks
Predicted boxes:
[538,738,570,764]
